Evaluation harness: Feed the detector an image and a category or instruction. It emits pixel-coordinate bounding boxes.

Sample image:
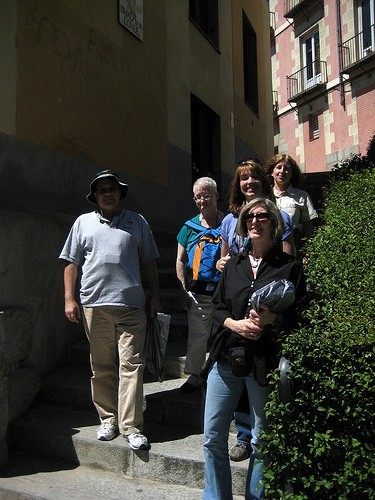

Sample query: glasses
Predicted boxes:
[192,193,215,203]
[243,212,271,222]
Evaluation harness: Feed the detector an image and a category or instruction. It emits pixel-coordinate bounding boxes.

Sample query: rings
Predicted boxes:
[250,333,253,336]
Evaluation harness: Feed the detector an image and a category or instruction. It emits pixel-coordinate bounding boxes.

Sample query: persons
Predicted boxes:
[204,197,310,500]
[59,170,161,450]
[264,152,319,253]
[215,160,298,461]
[176,176,228,394]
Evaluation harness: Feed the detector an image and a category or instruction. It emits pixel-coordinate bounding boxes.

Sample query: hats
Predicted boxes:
[86,169,129,206]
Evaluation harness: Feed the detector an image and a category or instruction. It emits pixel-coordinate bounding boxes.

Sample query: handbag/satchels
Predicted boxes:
[228,346,252,376]
[145,311,171,382]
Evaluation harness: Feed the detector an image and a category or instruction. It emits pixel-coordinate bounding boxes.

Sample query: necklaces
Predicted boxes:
[252,256,260,267]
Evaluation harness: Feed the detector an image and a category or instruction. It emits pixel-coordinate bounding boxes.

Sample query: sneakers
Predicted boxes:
[96,424,118,440]
[229,443,252,461]
[122,432,150,450]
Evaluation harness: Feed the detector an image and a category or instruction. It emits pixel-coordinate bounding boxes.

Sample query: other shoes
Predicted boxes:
[178,378,200,394]
